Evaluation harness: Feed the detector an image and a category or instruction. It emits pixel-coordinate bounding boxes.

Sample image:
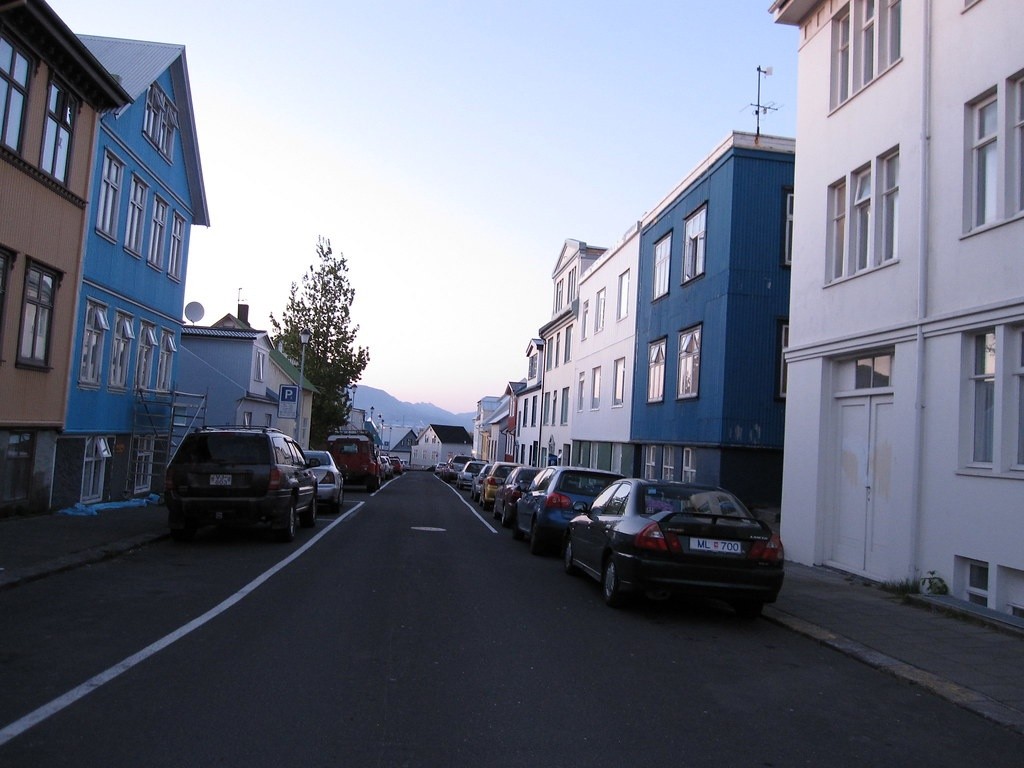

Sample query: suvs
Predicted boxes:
[163,426,321,541]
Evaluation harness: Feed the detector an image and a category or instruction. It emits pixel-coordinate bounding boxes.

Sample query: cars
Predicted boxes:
[564,477,784,618]
[471,464,492,502]
[493,467,543,526]
[456,461,489,488]
[302,451,348,514]
[514,465,626,553]
[479,462,519,510]
[379,455,411,481]
[435,455,477,483]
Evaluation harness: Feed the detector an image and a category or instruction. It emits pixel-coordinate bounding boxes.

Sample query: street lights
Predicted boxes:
[294,329,311,443]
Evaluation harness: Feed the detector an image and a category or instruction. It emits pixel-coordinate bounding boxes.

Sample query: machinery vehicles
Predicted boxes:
[327,430,381,494]
